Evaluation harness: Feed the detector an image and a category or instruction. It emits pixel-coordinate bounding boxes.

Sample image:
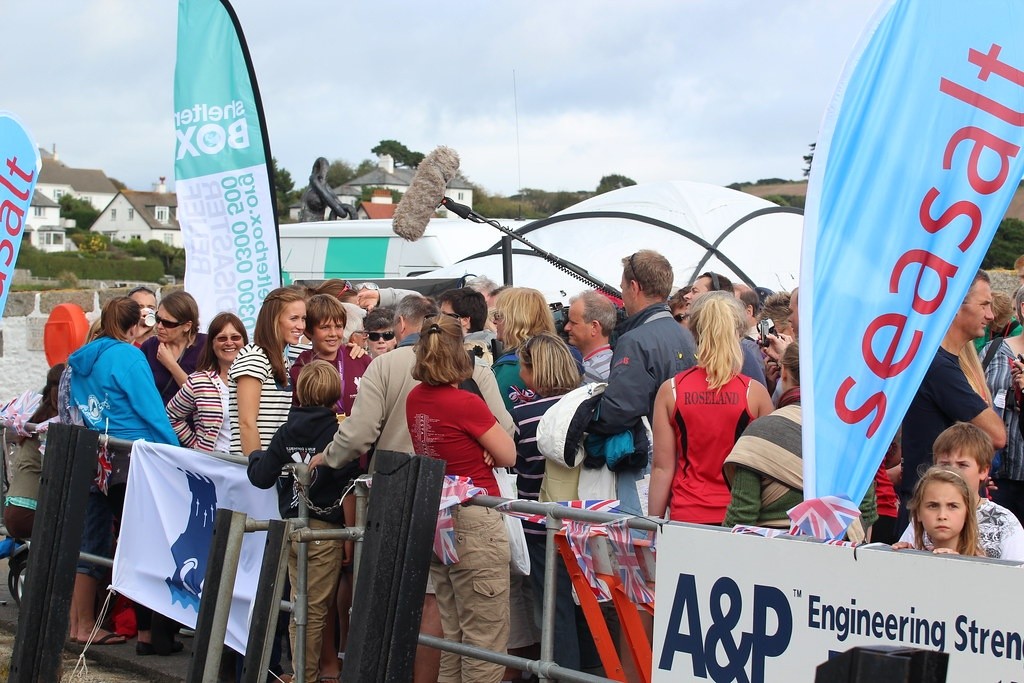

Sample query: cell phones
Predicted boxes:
[760,320,769,347]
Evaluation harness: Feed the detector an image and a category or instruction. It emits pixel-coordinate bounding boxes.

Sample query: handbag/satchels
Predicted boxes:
[492,467,531,576]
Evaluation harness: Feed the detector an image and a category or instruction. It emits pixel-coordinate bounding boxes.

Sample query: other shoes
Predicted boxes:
[179,627,194,635]
[132,638,184,655]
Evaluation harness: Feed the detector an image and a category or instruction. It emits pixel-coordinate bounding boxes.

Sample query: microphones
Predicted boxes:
[392,146,460,241]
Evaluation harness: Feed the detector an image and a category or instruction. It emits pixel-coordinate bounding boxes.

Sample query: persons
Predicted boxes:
[28,284,515,683]
[566,291,613,386]
[647,290,774,527]
[584,249,698,678]
[490,287,560,683]
[511,331,581,683]
[670,254,1024,562]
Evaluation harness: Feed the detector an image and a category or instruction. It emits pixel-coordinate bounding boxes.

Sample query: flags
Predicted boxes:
[433,475,861,605]
[175,0,283,344]
[0,389,113,497]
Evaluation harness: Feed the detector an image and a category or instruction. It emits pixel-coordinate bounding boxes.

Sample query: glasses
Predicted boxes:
[494,313,504,320]
[524,336,532,358]
[423,312,460,318]
[213,335,242,342]
[128,286,155,297]
[368,331,394,341]
[353,282,379,290]
[155,311,189,328]
[673,313,688,322]
[336,279,352,298]
[628,253,642,291]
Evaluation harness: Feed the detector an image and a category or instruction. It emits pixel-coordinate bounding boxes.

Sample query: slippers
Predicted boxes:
[68,631,128,645]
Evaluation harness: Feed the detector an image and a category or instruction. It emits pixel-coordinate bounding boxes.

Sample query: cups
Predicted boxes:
[141,308,156,327]
